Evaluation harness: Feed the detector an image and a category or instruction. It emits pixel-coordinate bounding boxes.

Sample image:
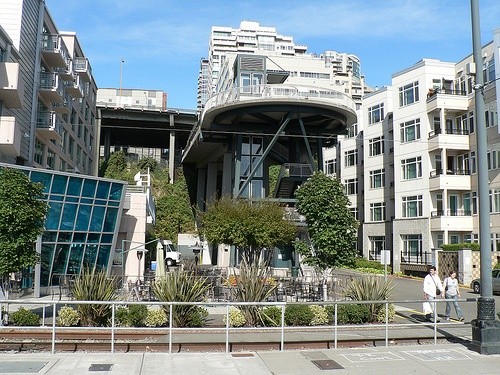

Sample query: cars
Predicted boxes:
[470,269,500,295]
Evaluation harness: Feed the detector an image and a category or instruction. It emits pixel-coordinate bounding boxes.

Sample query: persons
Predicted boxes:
[441,270,465,322]
[423,266,443,321]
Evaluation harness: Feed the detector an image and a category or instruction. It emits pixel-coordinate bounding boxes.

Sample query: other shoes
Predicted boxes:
[460,317,464,321]
[447,317,450,321]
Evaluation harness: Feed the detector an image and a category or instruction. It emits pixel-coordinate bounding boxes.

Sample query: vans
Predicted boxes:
[161,240,181,267]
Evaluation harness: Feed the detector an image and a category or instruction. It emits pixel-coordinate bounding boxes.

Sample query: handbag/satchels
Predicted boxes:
[436,289,440,295]
[421,298,431,313]
[445,278,448,291]
